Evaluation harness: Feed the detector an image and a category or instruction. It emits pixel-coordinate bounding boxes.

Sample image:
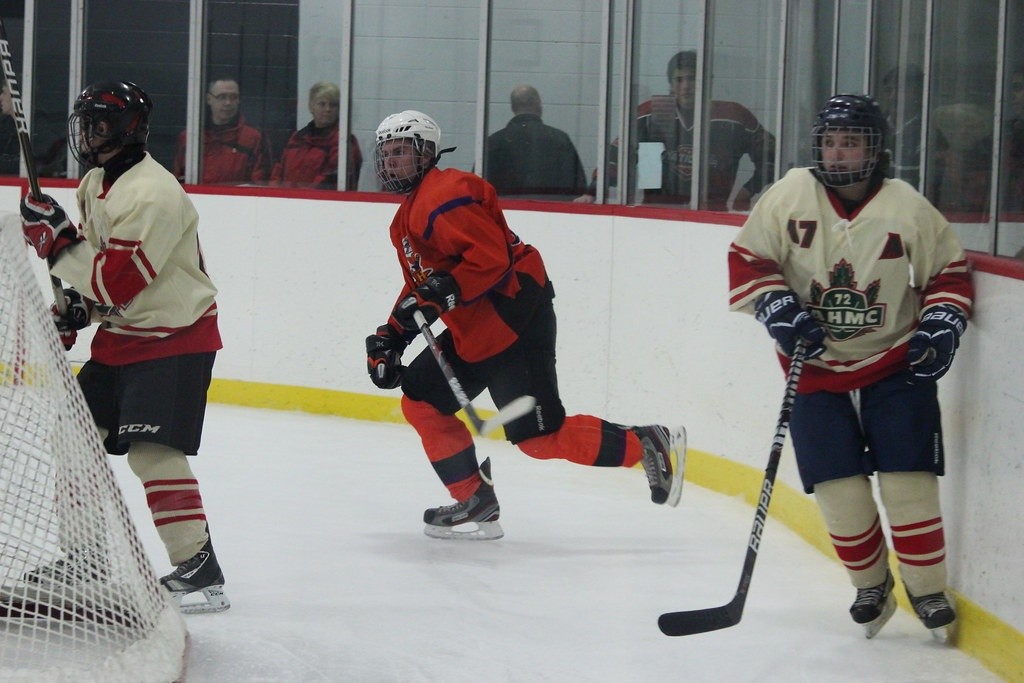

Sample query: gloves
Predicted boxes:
[50,286,95,351]
[754,290,826,361]
[366,334,402,389]
[19,194,77,259]
[394,272,461,334]
[906,306,968,387]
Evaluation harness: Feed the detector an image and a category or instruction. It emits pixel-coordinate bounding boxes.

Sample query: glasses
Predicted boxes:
[208,93,240,101]
[670,75,695,82]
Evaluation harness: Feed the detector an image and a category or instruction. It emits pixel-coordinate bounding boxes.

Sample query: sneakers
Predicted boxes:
[902,580,955,644]
[632,425,686,507]
[23,551,112,585]
[849,563,898,640]
[158,521,230,614]
[423,456,504,541]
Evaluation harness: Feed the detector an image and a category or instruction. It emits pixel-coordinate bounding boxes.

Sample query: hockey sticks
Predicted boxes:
[414,310,536,438]
[0,17,70,317]
[657,335,807,637]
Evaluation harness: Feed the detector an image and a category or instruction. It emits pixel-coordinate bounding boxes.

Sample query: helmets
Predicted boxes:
[374,109,457,195]
[67,78,154,169]
[810,93,887,187]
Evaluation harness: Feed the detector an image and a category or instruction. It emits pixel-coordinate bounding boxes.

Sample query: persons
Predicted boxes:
[728,93,974,646]
[471,85,587,193]
[1002,61,1024,214]
[270,83,363,191]
[173,75,262,184]
[366,110,686,539]
[572,51,775,212]
[879,64,949,210]
[20,79,230,612]
